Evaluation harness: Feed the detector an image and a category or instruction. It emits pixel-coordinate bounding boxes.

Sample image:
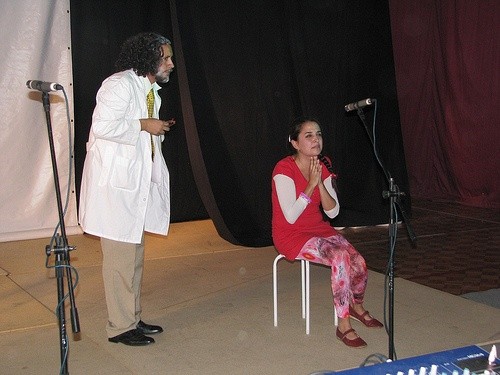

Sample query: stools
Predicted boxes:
[273,254,339,335]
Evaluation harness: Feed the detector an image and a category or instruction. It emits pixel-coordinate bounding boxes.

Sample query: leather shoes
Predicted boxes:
[136,320,164,335]
[108,329,155,346]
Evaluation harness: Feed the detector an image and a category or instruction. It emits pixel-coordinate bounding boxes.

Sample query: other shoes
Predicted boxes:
[348,303,384,328]
[336,326,368,349]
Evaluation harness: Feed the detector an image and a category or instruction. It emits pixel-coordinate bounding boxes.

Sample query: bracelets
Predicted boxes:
[300,192,312,202]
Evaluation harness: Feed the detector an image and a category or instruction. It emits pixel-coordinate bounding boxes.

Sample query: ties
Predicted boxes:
[147,88,155,162]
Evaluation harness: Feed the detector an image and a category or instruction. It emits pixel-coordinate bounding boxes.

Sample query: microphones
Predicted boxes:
[26,80,63,92]
[344,98,374,112]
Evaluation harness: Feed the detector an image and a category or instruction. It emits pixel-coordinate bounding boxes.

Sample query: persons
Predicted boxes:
[272,119,383,349]
[79,32,175,347]
